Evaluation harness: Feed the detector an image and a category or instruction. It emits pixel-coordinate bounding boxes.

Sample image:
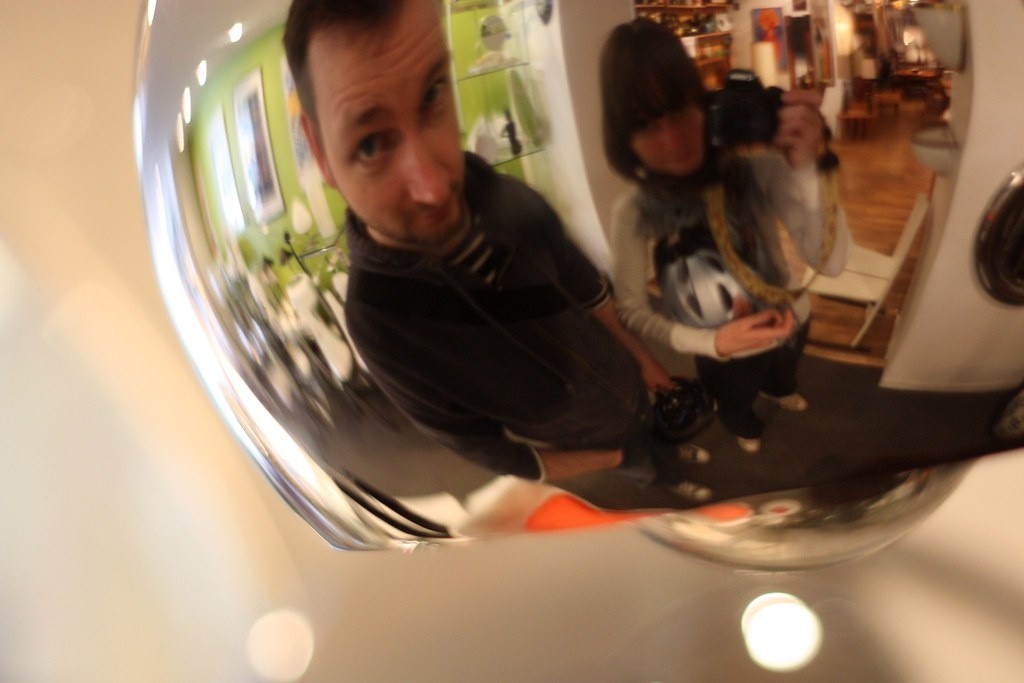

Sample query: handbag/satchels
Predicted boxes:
[655,376,713,439]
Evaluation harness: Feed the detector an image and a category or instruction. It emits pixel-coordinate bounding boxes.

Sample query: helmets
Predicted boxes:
[665,250,753,331]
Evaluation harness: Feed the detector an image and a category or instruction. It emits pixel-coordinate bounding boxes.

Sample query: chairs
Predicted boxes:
[809,192,930,354]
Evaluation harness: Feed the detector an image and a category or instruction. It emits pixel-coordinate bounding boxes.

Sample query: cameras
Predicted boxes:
[703,68,788,150]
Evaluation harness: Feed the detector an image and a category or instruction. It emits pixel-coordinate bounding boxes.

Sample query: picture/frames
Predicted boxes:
[204,104,248,239]
[281,57,327,195]
[230,65,287,226]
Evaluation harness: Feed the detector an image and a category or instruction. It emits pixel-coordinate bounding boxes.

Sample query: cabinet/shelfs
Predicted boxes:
[449,0,549,167]
[636,3,732,65]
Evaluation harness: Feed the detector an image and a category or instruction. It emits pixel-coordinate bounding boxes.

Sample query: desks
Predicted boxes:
[900,70,938,98]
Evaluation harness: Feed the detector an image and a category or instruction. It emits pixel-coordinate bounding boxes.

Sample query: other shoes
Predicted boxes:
[758,390,808,411]
[669,479,713,501]
[676,442,710,463]
[735,433,761,452]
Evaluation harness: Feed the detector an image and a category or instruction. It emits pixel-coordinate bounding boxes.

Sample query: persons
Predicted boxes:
[282,0,715,504]
[599,18,849,455]
[854,34,898,112]
